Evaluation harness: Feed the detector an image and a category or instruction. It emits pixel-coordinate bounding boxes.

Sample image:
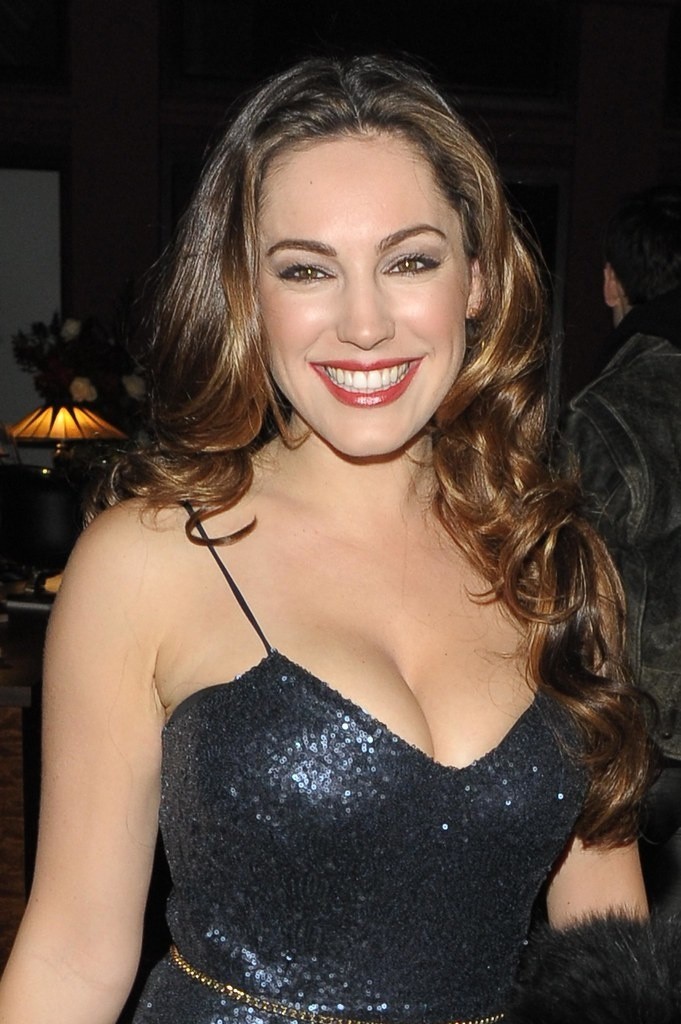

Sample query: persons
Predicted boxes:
[0,48,680,1024]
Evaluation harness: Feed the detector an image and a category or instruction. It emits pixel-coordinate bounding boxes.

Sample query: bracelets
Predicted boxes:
[23,570,48,595]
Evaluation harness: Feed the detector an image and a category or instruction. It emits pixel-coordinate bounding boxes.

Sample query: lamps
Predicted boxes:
[3,394,127,608]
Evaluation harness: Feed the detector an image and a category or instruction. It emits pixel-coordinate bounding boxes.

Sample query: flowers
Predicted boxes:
[11,317,142,410]
